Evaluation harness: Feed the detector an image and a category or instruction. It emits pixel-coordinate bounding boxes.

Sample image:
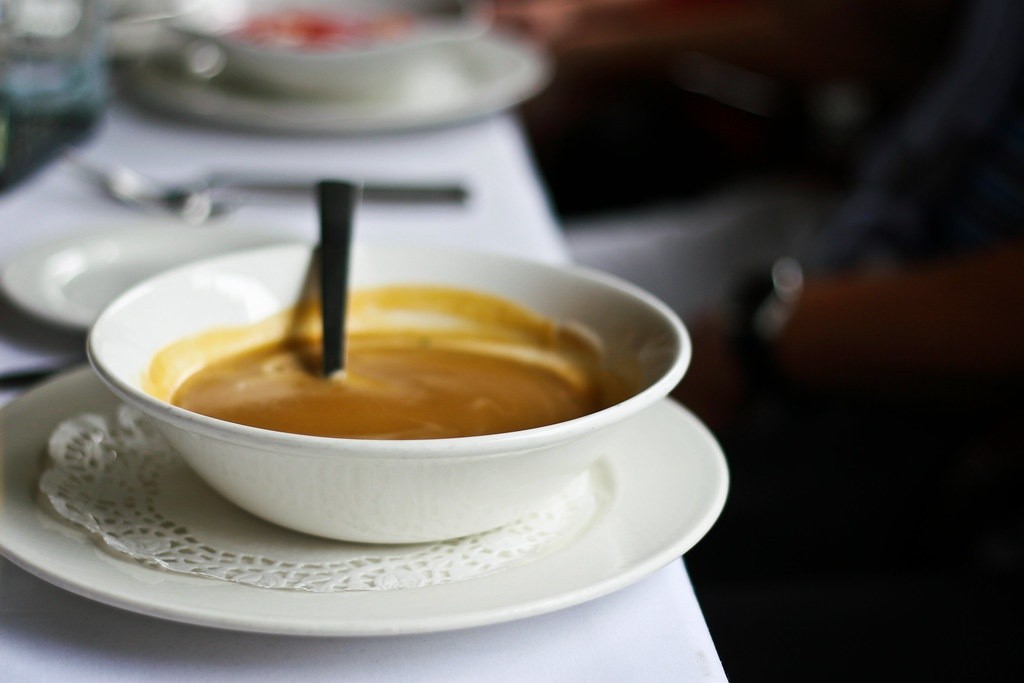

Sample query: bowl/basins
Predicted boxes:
[171,0,492,93]
[85,244,694,542]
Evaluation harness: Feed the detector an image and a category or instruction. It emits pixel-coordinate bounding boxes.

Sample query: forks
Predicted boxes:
[60,146,466,209]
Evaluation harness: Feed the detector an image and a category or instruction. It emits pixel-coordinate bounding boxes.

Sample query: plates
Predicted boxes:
[112,27,548,128]
[0,223,328,331]
[0,370,731,635]
